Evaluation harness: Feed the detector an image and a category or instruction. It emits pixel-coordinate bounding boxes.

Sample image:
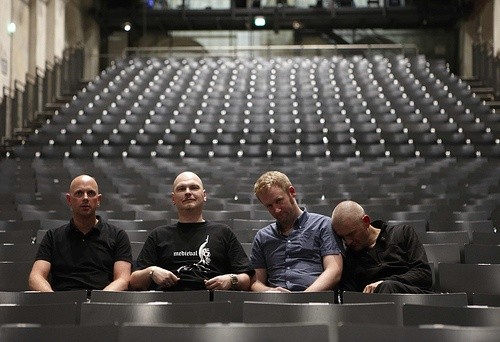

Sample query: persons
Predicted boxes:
[248,171,345,304]
[331,200,433,303]
[129,171,256,301]
[28,174,133,302]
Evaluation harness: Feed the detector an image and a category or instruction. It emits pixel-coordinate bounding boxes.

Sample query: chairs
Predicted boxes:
[0,52,500,342]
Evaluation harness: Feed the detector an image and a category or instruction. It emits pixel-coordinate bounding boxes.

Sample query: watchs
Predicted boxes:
[228,273,239,290]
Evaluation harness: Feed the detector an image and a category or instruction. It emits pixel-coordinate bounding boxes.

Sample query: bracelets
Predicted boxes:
[149,266,157,277]
